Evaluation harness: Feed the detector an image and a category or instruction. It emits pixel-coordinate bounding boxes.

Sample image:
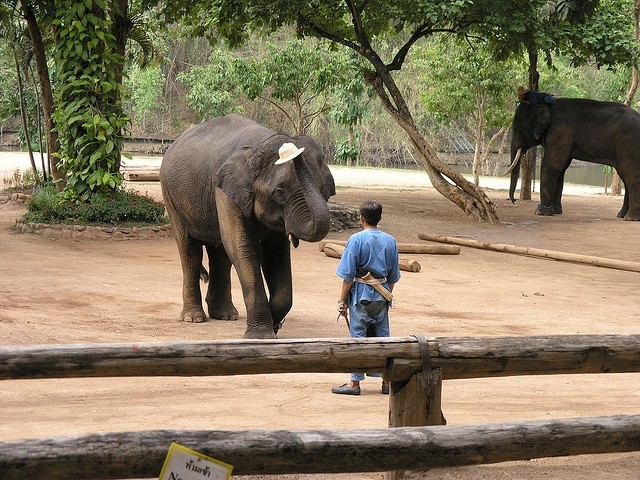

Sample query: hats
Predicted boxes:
[274,142,305,165]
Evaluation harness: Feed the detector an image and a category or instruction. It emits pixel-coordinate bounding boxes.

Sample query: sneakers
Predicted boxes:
[332,383,361,396]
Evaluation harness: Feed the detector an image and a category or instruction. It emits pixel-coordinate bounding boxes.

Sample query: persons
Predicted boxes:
[331,200,401,395]
[517,86,556,106]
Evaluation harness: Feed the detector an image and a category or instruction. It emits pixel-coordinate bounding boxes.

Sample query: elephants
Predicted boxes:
[158,111,337,343]
[504,91,640,223]
